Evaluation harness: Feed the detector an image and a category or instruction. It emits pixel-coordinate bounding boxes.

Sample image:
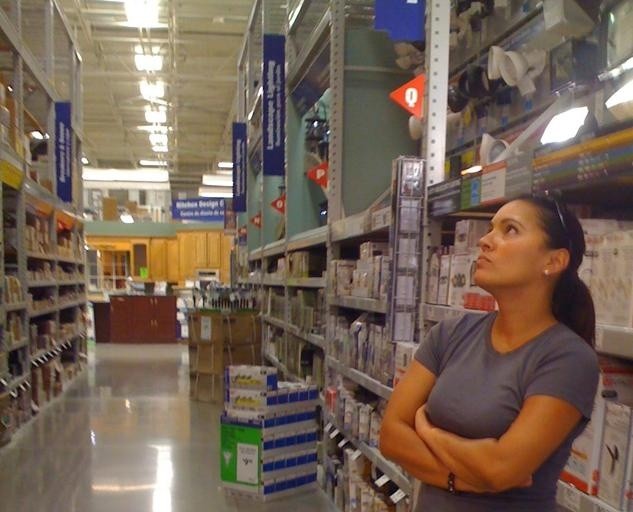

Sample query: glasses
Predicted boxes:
[533,188,568,232]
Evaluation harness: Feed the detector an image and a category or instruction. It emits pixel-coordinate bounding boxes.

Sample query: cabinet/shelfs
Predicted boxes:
[85,229,238,343]
[0,0,87,453]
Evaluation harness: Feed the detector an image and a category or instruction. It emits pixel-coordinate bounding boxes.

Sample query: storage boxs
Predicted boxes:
[187,307,262,405]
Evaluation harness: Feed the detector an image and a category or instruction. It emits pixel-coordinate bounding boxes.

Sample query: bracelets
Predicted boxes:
[445,470,459,498]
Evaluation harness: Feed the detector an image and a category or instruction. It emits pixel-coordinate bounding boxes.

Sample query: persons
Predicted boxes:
[379,189,603,511]
